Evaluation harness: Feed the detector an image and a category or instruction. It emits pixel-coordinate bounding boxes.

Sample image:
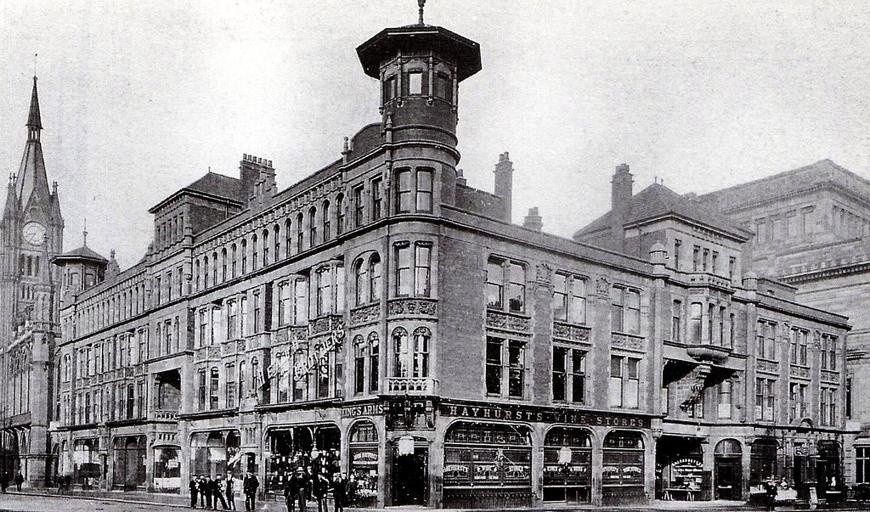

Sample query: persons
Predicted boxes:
[64,472,72,492]
[87,473,94,490]
[57,476,64,495]
[15,470,24,492]
[779,476,788,490]
[766,478,778,511]
[189,439,365,512]
[1,471,10,492]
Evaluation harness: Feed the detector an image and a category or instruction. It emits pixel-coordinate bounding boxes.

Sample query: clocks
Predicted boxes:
[22,221,48,246]
